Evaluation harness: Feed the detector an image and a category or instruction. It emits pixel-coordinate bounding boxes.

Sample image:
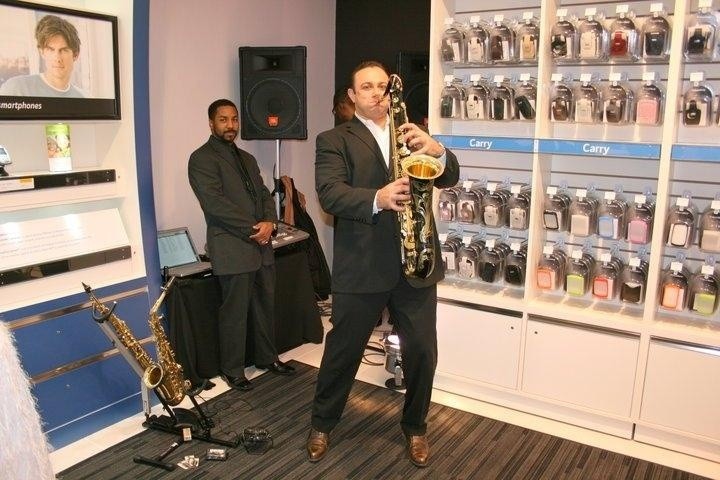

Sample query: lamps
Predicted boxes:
[383,335,405,375]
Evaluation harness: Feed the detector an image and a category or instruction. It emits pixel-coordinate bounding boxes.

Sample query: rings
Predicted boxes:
[266,241,268,243]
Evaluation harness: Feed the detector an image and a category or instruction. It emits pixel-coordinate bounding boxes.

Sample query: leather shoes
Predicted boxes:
[407,435,428,467]
[306,428,328,461]
[220,371,253,390]
[255,360,296,374]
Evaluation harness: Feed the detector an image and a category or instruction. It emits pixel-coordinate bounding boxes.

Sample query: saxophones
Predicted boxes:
[81,275,191,406]
[378,74,445,281]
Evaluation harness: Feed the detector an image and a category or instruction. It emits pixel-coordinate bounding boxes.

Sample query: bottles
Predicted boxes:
[45,124,73,172]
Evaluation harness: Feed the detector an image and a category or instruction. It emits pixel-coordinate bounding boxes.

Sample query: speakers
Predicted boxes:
[396,50,429,124]
[239,46,308,140]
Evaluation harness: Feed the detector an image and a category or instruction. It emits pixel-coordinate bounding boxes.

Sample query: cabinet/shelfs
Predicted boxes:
[0,1,162,453]
[427,0,720,479]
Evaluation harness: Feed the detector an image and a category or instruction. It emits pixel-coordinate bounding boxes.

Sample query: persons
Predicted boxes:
[328,88,382,330]
[304,61,459,468]
[0,14,86,99]
[187,99,295,391]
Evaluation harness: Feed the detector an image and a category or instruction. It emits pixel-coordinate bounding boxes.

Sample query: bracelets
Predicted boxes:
[272,221,278,231]
[434,140,445,157]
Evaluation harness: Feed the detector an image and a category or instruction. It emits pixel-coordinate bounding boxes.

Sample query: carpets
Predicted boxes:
[56,360,711,478]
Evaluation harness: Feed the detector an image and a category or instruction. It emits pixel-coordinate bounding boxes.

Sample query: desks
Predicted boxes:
[161,251,324,390]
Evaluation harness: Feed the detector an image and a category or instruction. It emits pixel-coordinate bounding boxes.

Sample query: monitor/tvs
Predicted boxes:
[0,0,121,120]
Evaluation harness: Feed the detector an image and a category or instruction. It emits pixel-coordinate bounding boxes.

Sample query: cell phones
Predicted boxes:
[0,144,12,166]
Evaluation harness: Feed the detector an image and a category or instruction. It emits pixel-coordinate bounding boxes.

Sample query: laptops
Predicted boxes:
[157,227,213,277]
